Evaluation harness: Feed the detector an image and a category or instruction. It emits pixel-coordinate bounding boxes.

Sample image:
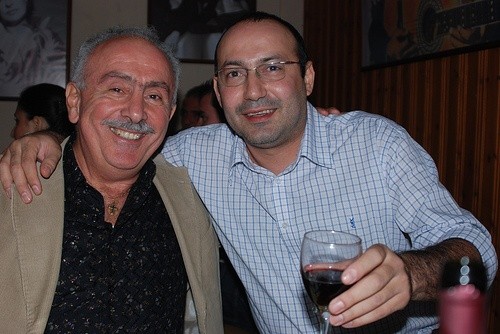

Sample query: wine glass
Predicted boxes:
[300,231,362,334]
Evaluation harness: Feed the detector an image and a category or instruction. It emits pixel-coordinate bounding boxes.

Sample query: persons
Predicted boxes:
[1,27,225,333]
[0,11,498,334]
[174,82,231,130]
[10,84,72,141]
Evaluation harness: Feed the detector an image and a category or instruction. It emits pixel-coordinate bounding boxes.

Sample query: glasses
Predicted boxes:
[214,61,302,87]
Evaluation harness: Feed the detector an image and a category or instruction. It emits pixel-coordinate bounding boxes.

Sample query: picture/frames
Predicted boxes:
[0,0,73,102]
[146,0,258,65]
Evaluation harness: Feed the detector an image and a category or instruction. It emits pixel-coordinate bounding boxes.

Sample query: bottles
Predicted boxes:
[432,258,487,333]
